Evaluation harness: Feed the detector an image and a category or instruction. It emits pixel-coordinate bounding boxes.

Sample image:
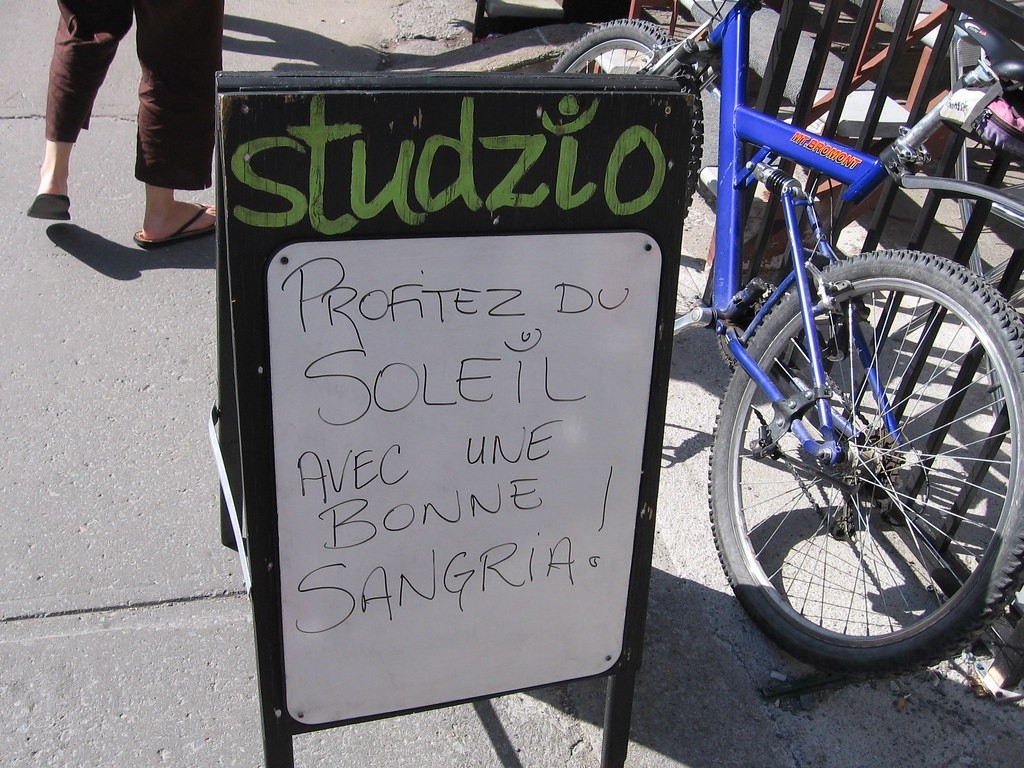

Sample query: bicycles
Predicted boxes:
[542,0,1024,670]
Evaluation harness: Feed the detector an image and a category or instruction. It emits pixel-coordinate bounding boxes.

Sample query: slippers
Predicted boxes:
[133,202,215,249]
[27,193,71,220]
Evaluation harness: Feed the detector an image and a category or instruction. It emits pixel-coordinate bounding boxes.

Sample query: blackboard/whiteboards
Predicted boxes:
[216,70,694,768]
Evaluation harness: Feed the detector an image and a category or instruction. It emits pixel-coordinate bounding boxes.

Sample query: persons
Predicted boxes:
[28,0,224,248]
